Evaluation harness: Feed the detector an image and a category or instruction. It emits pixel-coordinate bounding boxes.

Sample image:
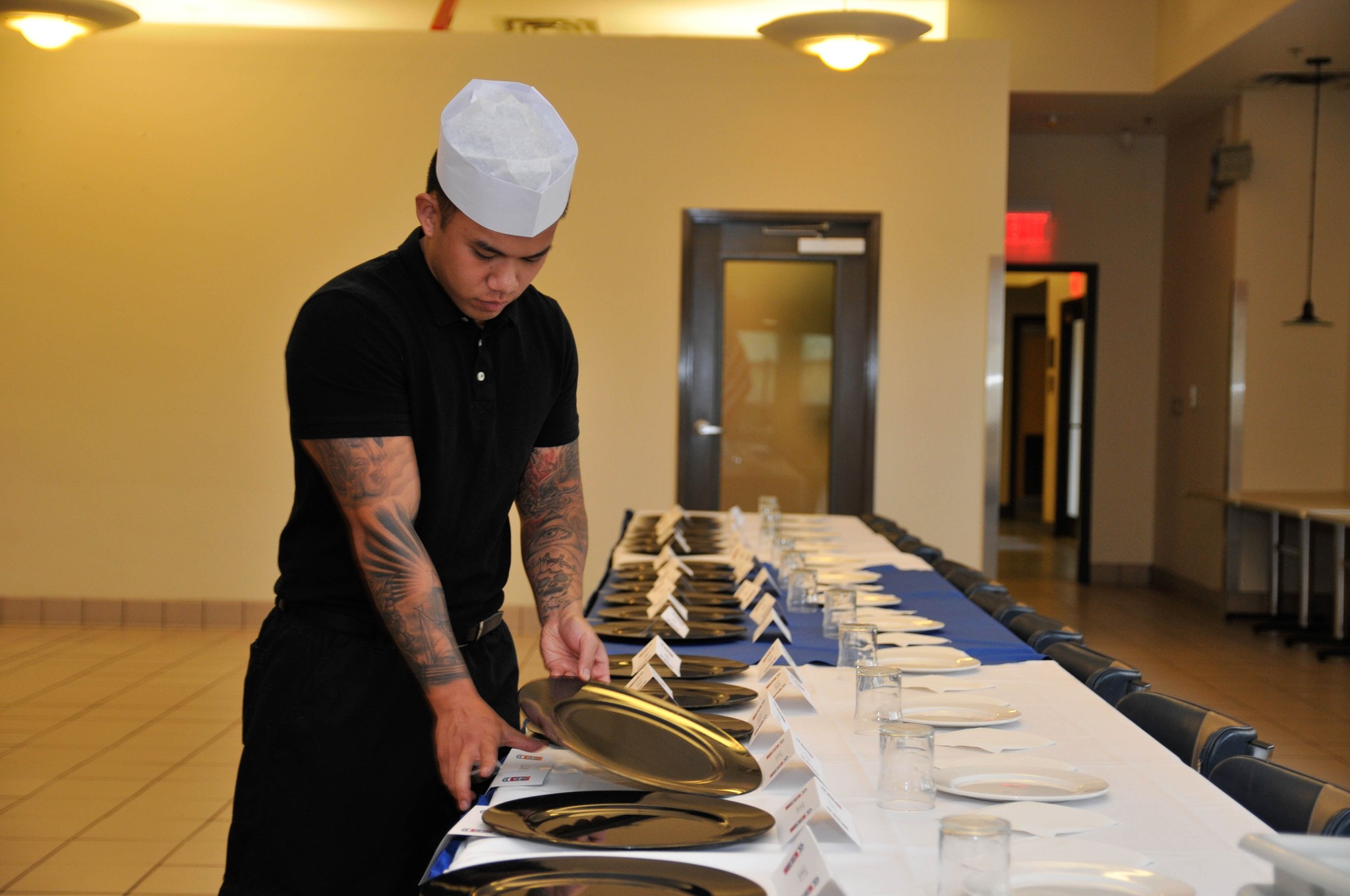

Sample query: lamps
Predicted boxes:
[1282,55,1335,328]
[758,11,932,71]
[0,0,140,51]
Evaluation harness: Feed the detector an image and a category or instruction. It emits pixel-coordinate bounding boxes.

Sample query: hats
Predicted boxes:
[436,78,580,236]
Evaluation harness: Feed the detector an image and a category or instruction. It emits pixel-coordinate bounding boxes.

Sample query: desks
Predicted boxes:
[1192,491,1350,663]
[422,510,1280,896]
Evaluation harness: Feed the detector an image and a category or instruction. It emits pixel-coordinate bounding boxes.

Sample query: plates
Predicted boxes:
[856,656,981,672]
[416,856,767,895]
[1011,861,1193,895]
[765,513,881,585]
[877,699,1021,727]
[518,676,761,797]
[625,515,726,555]
[591,560,747,641]
[838,615,945,632]
[934,755,1110,803]
[605,654,749,676]
[482,790,775,849]
[809,593,900,607]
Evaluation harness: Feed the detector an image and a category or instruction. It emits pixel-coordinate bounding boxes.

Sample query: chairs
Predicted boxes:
[1209,754,1350,837]
[861,514,1036,621]
[1005,613,1084,652]
[1116,691,1274,777]
[1042,644,1150,695]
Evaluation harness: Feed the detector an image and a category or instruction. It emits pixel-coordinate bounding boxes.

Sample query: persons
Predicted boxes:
[217,80,610,896]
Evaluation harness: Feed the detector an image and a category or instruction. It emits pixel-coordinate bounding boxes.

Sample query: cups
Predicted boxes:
[837,623,878,674]
[823,587,857,641]
[786,569,818,614]
[853,666,902,736]
[937,814,1011,895]
[877,723,934,810]
[757,495,804,590]
